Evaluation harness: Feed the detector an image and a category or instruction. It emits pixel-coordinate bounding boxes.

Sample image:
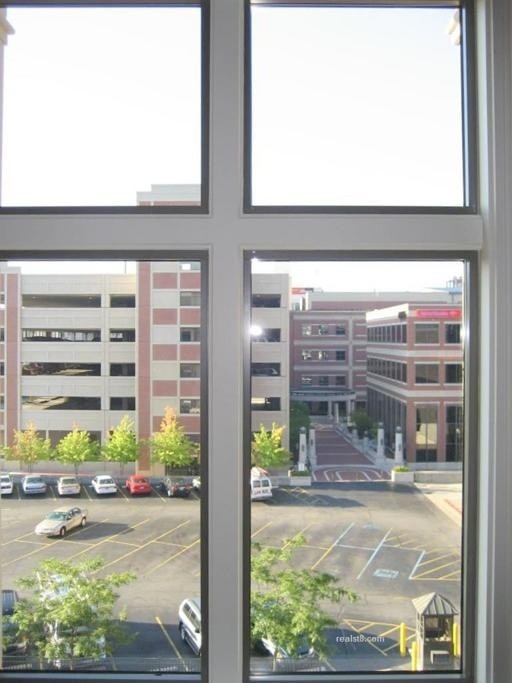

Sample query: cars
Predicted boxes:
[20,474,47,494]
[91,474,118,495]
[126,474,151,495]
[56,476,80,496]
[192,475,201,491]
[159,476,191,496]
[0,588,30,657]
[0,474,13,494]
[34,505,88,537]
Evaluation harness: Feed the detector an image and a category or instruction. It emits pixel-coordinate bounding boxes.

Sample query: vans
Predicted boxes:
[177,597,201,657]
[35,569,107,668]
[250,474,272,500]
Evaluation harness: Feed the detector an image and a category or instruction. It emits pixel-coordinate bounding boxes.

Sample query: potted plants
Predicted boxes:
[288,469,311,486]
[390,466,415,483]
